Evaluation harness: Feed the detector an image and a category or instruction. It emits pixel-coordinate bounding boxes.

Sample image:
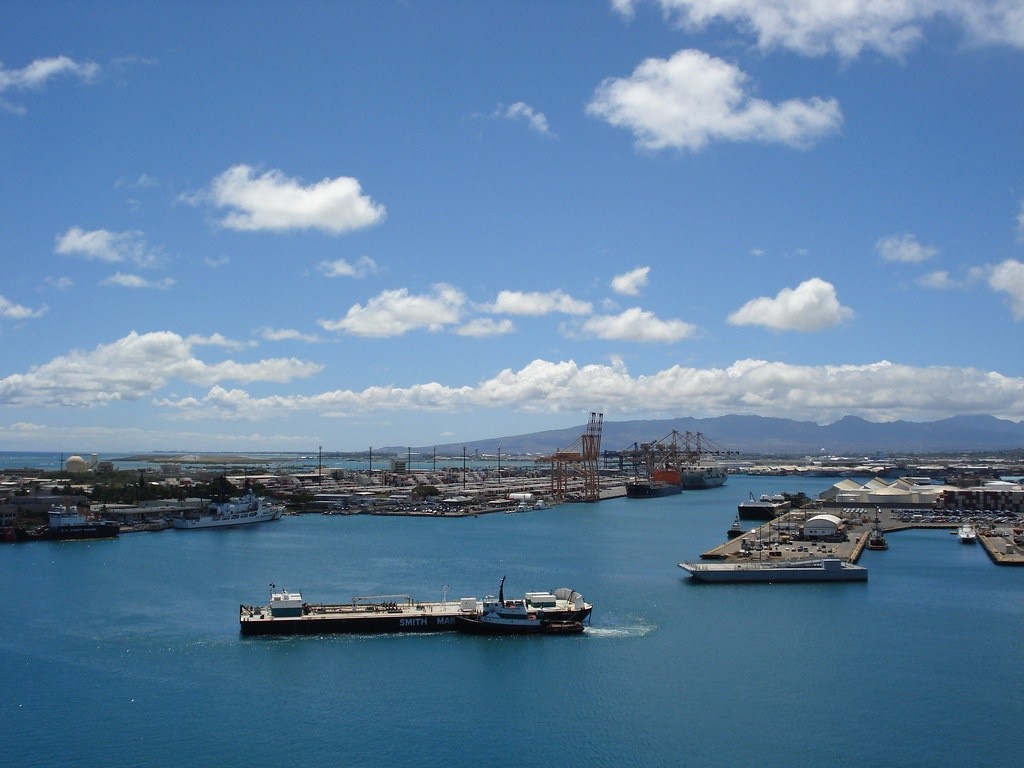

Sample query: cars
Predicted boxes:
[842,508,868,515]
[980,524,1010,538]
[888,509,1024,524]
[755,540,832,555]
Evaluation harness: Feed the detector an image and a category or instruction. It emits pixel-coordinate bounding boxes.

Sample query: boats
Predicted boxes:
[737,491,811,519]
[504,499,553,514]
[454,613,585,635]
[677,553,869,582]
[958,524,977,542]
[173,492,285,530]
[866,525,888,550]
[239,575,594,636]
[624,480,682,498]
[726,516,746,538]
[39,504,122,541]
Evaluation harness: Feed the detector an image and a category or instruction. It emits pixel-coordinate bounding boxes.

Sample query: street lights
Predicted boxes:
[368,446,373,477]
[318,445,322,487]
[407,447,411,475]
[433,447,436,472]
[463,446,466,488]
[498,446,500,484]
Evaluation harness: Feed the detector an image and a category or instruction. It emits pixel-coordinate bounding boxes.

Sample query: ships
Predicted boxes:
[647,429,728,489]
